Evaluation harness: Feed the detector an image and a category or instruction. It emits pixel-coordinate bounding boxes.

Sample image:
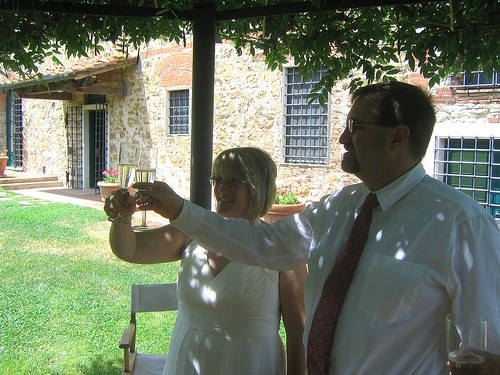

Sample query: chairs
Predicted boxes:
[119,282,178,375]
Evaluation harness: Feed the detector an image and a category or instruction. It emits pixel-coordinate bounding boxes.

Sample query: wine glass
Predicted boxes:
[129,146,158,234]
[107,142,142,224]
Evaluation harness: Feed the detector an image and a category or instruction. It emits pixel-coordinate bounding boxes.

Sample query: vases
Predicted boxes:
[0,155,9,175]
[263,203,305,224]
[97,181,120,201]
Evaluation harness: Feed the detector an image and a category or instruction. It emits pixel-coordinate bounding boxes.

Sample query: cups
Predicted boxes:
[445,311,488,375]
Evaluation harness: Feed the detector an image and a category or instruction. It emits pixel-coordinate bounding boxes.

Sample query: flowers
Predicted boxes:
[274,190,299,205]
[102,169,119,183]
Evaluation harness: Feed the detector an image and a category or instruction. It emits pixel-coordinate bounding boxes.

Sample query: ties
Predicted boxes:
[306,191,379,375]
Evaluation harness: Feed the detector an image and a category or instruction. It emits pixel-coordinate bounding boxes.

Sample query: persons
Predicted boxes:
[132,81,500,375]
[104,147,308,375]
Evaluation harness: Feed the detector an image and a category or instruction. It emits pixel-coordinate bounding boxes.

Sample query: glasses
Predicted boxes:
[209,175,247,189]
[342,118,387,135]
